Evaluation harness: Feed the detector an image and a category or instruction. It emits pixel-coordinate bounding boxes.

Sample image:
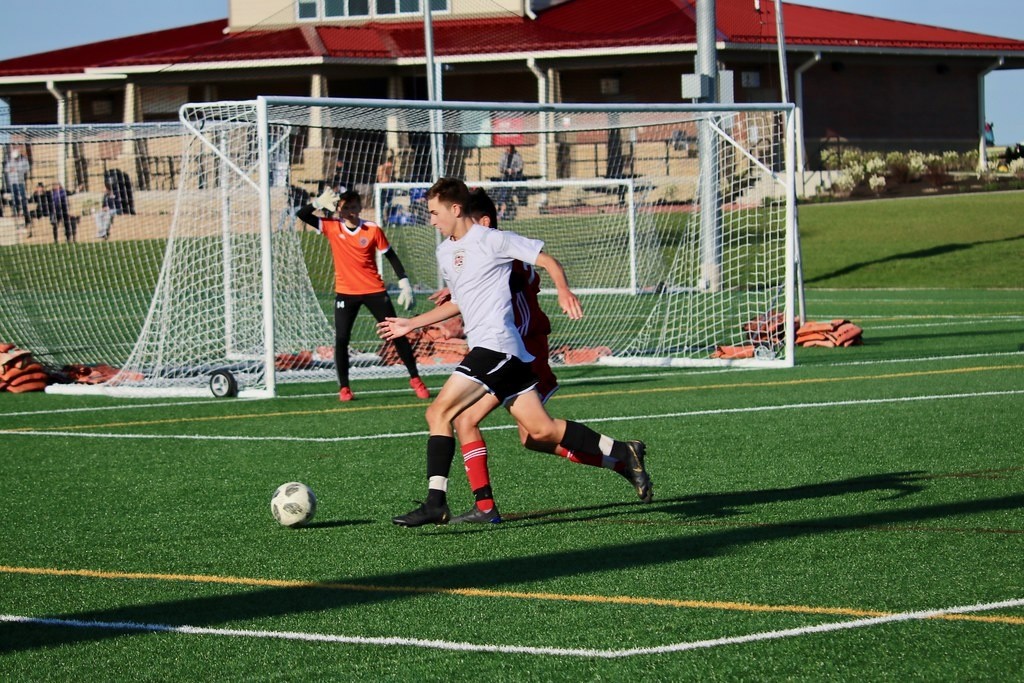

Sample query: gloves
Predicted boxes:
[397,278,417,309]
[312,186,340,212]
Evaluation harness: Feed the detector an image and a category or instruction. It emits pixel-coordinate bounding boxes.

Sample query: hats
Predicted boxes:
[12,150,20,158]
[37,182,43,187]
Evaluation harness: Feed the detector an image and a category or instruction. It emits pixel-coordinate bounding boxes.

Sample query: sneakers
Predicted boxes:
[619,441,649,498]
[620,462,655,504]
[449,503,501,524]
[339,388,354,402]
[391,499,449,526]
[410,376,431,398]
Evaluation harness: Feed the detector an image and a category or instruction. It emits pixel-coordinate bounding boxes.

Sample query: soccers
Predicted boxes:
[270,482,317,529]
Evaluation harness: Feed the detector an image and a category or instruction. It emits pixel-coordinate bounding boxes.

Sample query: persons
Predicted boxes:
[374,177,652,519]
[277,142,524,231]
[0,149,123,243]
[295,187,430,402]
